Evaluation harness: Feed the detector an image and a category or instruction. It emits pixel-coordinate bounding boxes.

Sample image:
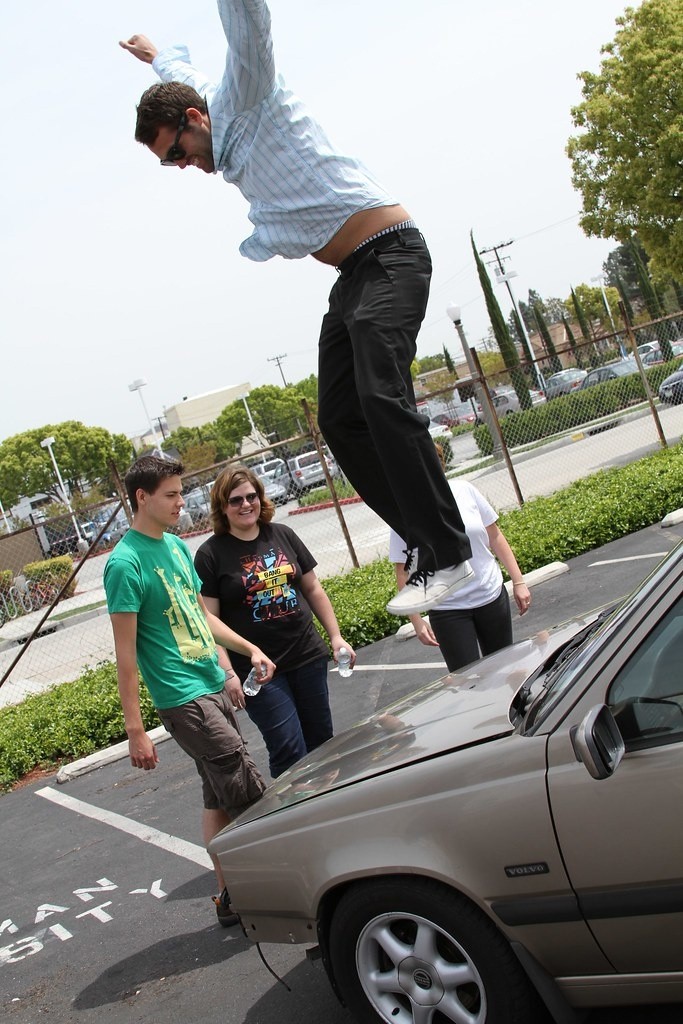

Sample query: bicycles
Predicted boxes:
[23,573,64,611]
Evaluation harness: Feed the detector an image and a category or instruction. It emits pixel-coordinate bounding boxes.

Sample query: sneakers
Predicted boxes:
[402,547,419,580]
[212,892,239,926]
[386,559,476,616]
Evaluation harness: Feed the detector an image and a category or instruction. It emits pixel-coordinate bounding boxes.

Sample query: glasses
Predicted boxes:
[160,106,192,166]
[227,492,259,507]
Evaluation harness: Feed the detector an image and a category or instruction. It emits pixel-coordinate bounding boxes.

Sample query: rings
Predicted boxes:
[526,602,530,605]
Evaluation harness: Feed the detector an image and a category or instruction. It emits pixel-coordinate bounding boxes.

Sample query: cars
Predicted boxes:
[628,339,683,365]
[427,401,483,443]
[540,368,587,400]
[658,363,683,405]
[570,359,652,394]
[209,545,683,1024]
[475,390,548,429]
[81,471,288,551]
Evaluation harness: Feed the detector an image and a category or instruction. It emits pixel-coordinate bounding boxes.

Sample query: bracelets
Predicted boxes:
[513,581,526,586]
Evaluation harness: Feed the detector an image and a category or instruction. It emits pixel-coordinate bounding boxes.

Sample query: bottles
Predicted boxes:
[243,664,266,697]
[336,646,353,678]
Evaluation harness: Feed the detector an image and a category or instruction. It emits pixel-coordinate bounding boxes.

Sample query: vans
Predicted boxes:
[269,450,334,497]
[249,459,285,477]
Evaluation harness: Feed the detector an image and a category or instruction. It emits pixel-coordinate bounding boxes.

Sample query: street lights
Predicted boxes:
[40,437,90,553]
[496,272,553,401]
[448,305,512,459]
[236,390,267,464]
[590,274,631,359]
[128,377,167,459]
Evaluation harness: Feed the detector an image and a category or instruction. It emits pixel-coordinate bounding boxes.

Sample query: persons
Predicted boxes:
[390,444,533,677]
[103,456,277,926]
[119,0,476,615]
[194,465,357,779]
